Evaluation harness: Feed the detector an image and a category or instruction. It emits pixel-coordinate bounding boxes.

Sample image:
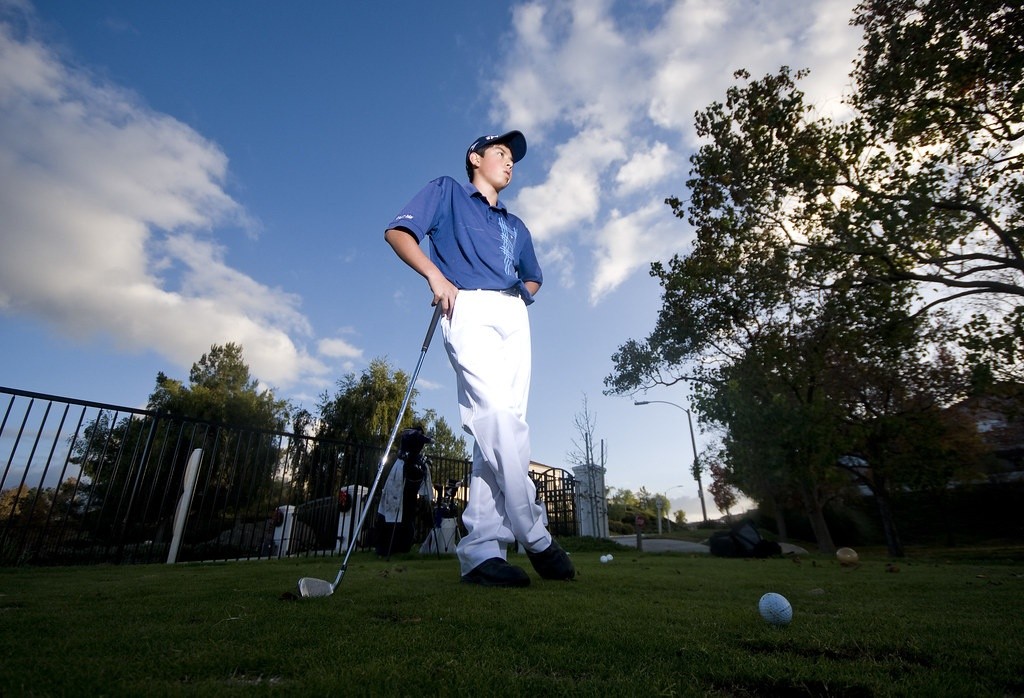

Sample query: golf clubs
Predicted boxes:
[296,303,445,597]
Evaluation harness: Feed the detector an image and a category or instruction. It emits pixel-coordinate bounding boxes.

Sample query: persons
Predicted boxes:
[384,130,576,587]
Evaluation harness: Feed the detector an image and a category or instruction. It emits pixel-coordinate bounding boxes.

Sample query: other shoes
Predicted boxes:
[526,535,575,582]
[461,558,531,588]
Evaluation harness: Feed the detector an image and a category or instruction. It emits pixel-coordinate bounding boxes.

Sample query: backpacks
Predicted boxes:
[710,518,782,559]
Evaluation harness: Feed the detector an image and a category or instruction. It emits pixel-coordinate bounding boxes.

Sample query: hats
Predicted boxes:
[466,130,527,171]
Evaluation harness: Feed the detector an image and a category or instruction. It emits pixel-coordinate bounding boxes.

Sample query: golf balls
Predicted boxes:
[601,555,608,564]
[759,593,794,626]
[607,554,613,561]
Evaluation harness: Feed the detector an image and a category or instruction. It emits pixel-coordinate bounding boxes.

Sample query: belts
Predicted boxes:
[503,287,519,298]
